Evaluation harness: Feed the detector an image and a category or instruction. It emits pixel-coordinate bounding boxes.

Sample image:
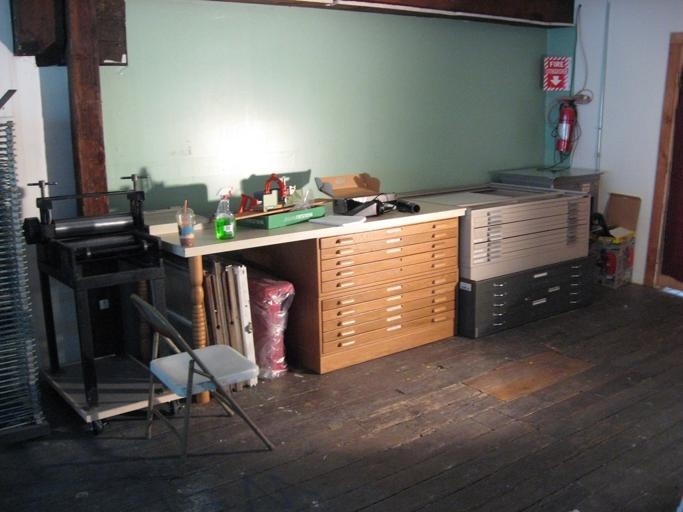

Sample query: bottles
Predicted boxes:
[214,199,236,240]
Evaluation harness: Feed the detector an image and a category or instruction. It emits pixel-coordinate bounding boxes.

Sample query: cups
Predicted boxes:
[175,207,197,248]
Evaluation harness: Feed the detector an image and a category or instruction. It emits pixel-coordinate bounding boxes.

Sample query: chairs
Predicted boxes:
[128,293,276,464]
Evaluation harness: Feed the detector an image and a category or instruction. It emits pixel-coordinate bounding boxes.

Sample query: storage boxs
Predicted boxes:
[590,193,641,291]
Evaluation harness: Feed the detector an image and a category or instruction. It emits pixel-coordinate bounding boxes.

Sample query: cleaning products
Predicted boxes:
[215,186,237,238]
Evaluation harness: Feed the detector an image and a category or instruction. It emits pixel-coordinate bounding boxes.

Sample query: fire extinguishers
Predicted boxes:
[557,99,577,154]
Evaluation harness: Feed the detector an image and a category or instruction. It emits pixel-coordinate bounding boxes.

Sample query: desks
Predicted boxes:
[145,209,467,260]
